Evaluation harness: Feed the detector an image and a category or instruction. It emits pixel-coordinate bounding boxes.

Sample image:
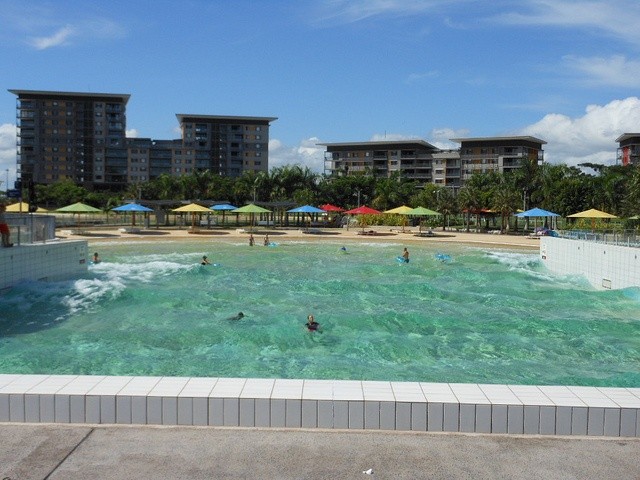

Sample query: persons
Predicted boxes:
[305,315,320,332]
[402,247,410,264]
[92,252,101,263]
[200,255,210,265]
[263,233,269,246]
[248,233,256,245]
[226,311,245,322]
[0,201,13,248]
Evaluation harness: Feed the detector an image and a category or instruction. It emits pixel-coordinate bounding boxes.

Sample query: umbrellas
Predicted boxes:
[53,202,103,233]
[209,204,238,223]
[399,205,443,233]
[566,208,620,233]
[513,207,561,236]
[171,202,214,234]
[286,204,326,230]
[4,201,48,212]
[230,203,273,227]
[321,203,347,212]
[382,205,413,231]
[343,205,382,232]
[110,203,154,225]
